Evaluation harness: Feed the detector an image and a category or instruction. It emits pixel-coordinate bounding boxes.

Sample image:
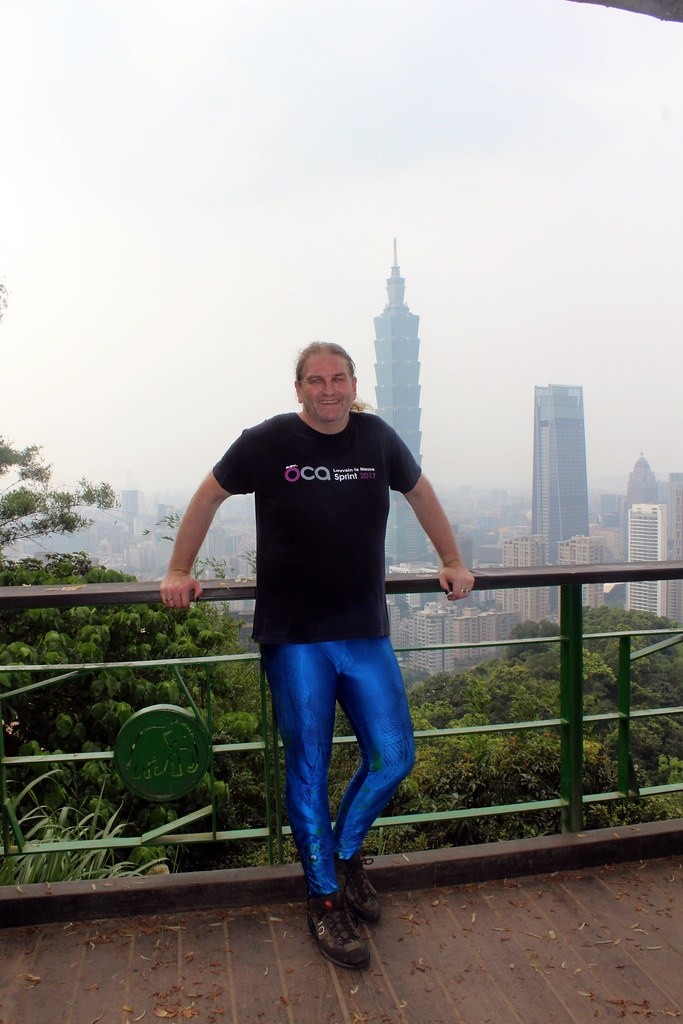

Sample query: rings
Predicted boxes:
[460,589,465,591]
[466,589,469,591]
[166,597,173,601]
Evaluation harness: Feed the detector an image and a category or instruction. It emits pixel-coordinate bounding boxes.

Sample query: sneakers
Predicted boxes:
[307,892,371,968]
[335,853,382,923]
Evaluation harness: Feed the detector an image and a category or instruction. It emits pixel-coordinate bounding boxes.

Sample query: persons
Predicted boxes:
[158,341,475,969]
